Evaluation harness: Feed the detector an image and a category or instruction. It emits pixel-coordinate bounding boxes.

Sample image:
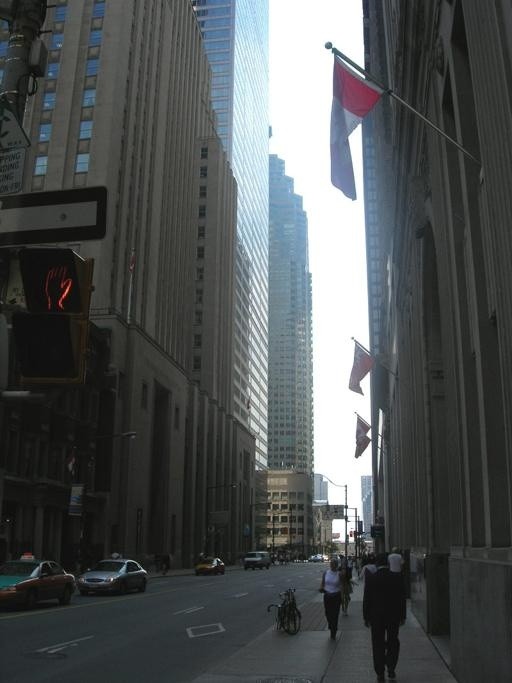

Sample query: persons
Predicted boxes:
[320,548,410,683]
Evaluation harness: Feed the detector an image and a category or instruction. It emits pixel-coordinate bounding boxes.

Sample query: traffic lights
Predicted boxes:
[12,242,88,384]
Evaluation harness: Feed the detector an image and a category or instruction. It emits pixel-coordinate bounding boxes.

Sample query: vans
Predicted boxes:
[243,550,273,570]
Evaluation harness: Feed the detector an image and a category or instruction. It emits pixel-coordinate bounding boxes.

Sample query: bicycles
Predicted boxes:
[274,584,302,634]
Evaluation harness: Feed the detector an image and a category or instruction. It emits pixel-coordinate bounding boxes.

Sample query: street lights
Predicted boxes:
[78,428,138,567]
[205,481,238,558]
[248,500,272,552]
[310,471,348,563]
[346,520,361,561]
[272,511,291,556]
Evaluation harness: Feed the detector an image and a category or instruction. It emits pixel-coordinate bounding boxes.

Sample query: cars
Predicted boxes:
[196,556,225,576]
[309,551,345,563]
[0,551,77,609]
[78,557,148,596]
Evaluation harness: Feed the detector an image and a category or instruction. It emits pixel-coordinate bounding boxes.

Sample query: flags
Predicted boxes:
[355,436,370,458]
[329,55,383,198]
[349,344,374,394]
[356,417,370,443]
